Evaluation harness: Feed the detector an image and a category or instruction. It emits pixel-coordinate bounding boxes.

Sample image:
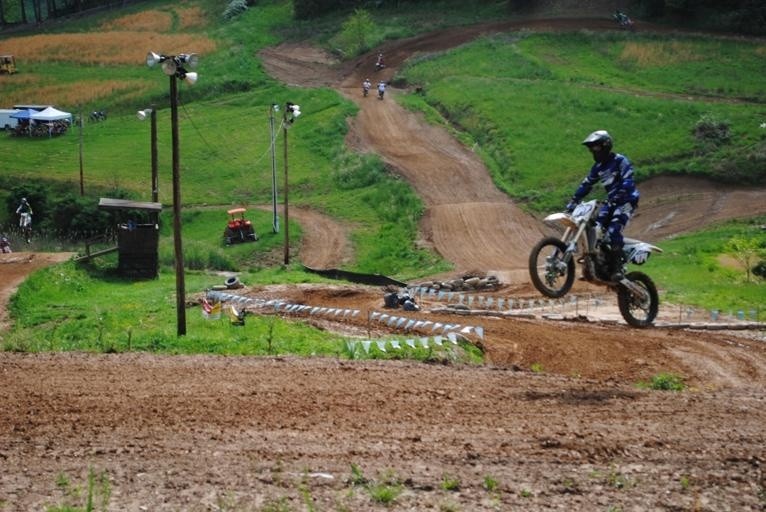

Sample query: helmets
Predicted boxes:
[20,198,27,204]
[580,130,614,163]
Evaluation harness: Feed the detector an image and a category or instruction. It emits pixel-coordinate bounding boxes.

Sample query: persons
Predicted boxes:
[16,197,33,231]
[615,10,628,26]
[377,80,387,97]
[375,53,385,69]
[563,130,639,281]
[363,78,371,96]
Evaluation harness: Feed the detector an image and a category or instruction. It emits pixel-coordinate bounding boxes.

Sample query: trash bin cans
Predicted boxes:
[117,222,158,279]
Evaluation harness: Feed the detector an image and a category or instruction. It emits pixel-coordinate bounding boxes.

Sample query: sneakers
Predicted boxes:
[578,276,587,281]
[611,270,625,281]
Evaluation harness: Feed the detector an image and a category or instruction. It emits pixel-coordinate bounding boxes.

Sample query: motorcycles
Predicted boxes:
[15,110,107,138]
[528,196,664,328]
[362,77,386,100]
[374,52,387,71]
[15,209,34,243]
